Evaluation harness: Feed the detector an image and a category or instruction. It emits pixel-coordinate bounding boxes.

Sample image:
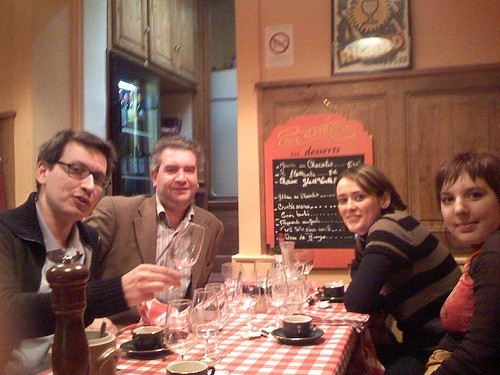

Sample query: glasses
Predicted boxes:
[52,160,111,190]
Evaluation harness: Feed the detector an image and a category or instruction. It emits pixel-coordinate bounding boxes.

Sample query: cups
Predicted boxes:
[166,361,216,375]
[131,325,164,350]
[282,314,313,337]
[84,329,119,375]
[323,283,345,297]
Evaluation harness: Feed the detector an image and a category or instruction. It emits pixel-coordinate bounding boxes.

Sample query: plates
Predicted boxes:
[320,293,346,302]
[119,340,169,356]
[272,326,324,344]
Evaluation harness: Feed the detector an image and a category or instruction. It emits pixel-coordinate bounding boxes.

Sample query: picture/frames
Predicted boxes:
[331,0,412,76]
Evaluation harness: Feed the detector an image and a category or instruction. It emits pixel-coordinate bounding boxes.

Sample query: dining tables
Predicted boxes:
[33,283,384,375]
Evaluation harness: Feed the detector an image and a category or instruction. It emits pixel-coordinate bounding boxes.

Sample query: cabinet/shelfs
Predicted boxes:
[132,0,196,95]
[109,0,151,84]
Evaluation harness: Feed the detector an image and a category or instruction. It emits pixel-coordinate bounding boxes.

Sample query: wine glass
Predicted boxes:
[162,220,315,367]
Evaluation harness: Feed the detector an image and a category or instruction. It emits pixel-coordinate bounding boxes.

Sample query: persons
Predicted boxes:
[425,151,500,375]
[83,134,225,334]
[0,127,182,375]
[317,168,463,374]
